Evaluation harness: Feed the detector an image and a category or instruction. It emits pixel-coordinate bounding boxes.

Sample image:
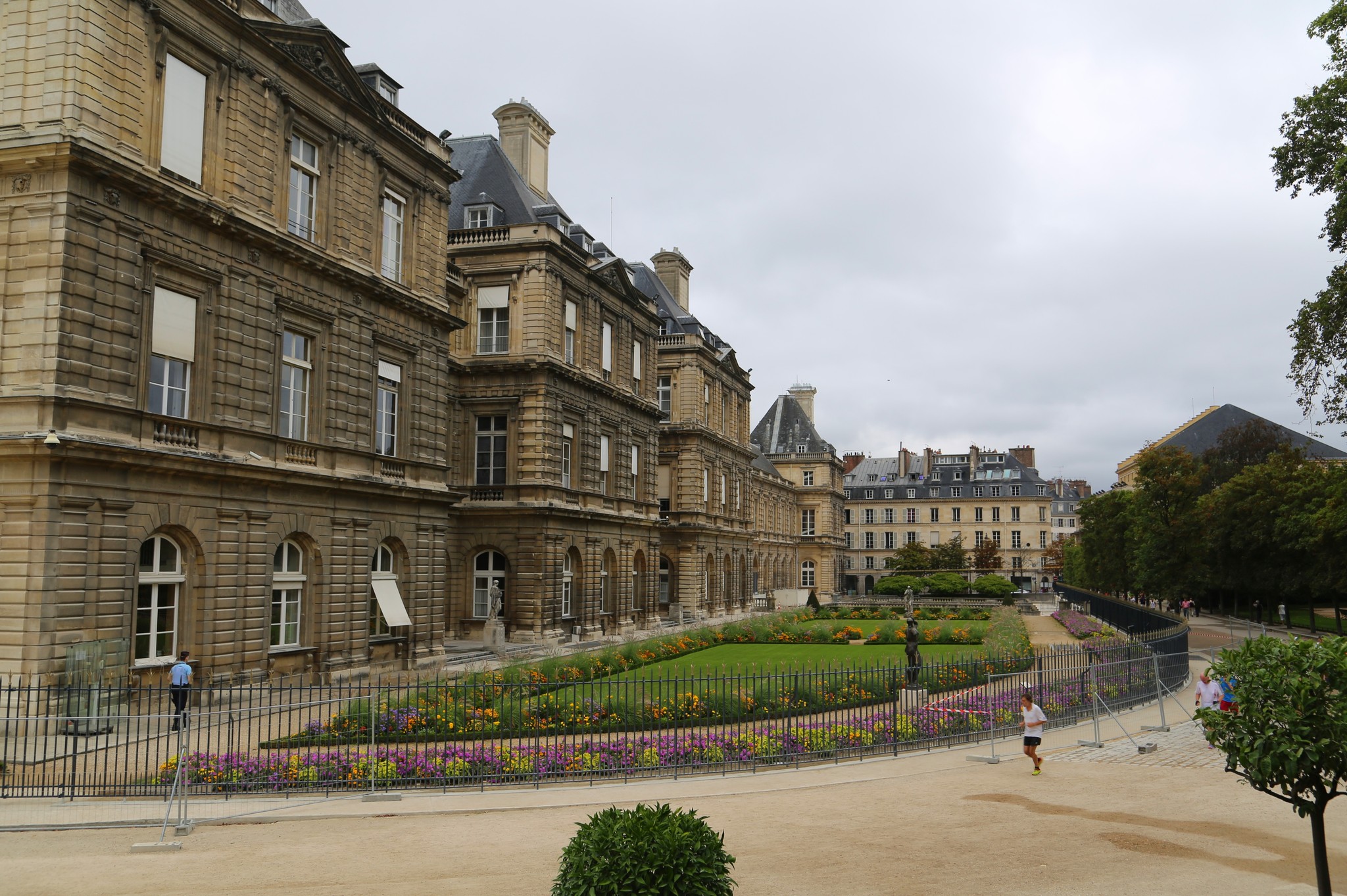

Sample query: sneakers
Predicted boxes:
[1208,743,1215,749]
[1037,757,1043,766]
[1031,768,1042,775]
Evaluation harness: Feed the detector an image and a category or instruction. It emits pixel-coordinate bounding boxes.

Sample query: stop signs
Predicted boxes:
[1053,576,1058,581]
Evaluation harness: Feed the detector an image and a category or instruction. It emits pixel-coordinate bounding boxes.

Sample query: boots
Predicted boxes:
[183,717,192,728]
[172,720,184,731]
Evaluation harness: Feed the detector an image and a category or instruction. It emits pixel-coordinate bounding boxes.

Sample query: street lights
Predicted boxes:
[968,555,972,596]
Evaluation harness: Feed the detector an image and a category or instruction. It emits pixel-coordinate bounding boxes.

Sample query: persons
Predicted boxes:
[1019,694,1048,775]
[1218,673,1239,718]
[1089,587,1202,621]
[904,617,922,686]
[488,579,501,620]
[1195,671,1224,749]
[1253,600,1262,624]
[904,586,914,615]
[166,650,194,731]
[1277,601,1286,626]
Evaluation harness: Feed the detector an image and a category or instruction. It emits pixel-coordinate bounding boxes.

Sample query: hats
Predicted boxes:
[180,651,189,656]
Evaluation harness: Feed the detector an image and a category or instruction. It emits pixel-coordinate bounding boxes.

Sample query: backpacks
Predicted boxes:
[1189,600,1194,608]
